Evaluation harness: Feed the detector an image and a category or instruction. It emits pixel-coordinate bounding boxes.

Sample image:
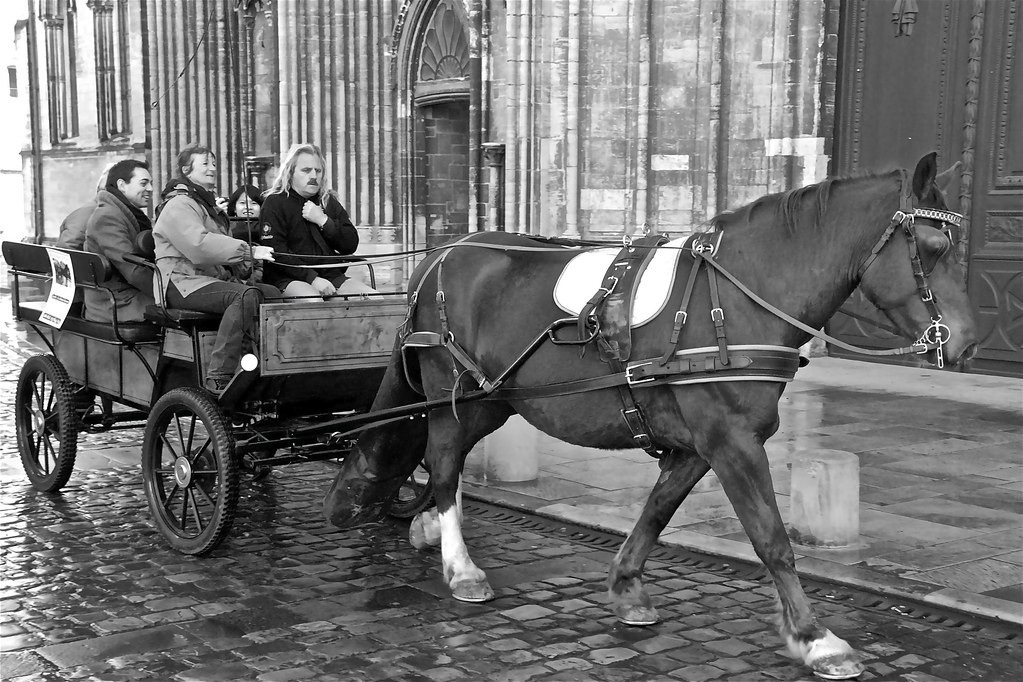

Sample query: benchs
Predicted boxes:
[122,222,375,326]
[1,240,154,345]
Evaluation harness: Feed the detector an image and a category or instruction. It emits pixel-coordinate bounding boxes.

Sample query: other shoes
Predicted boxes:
[205,377,230,394]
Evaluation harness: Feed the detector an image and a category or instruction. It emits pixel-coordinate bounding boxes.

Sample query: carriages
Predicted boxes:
[1,151,978,680]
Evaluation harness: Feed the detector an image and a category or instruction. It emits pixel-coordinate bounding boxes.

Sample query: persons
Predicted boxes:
[259,144,384,303]
[55,160,156,326]
[227,185,265,283]
[152,146,283,394]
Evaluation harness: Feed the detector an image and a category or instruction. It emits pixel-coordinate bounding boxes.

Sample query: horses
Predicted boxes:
[406,151,980,681]
